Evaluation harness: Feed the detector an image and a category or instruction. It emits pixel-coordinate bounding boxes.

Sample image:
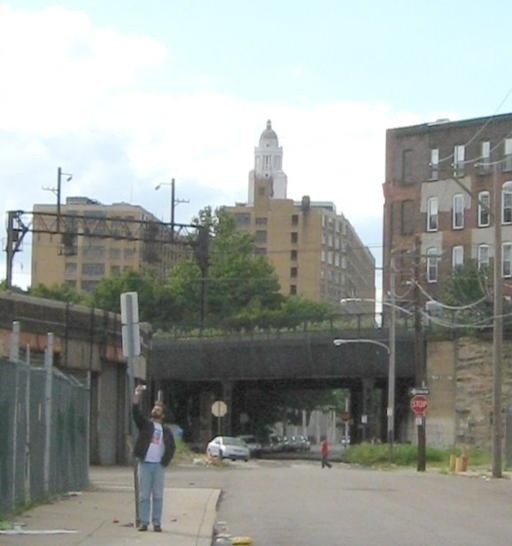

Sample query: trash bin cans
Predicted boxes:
[450,455,468,472]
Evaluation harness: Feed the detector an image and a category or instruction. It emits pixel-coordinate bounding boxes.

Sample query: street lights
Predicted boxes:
[340,297,426,472]
[333,338,396,470]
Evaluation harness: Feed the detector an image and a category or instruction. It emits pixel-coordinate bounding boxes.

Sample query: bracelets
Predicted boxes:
[134,390,141,396]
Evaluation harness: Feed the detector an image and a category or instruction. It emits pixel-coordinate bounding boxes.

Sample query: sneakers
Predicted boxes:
[154,526,162,533]
[137,524,147,532]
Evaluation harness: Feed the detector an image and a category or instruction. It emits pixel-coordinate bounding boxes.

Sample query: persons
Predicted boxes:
[132,384,176,532]
[320,434,333,470]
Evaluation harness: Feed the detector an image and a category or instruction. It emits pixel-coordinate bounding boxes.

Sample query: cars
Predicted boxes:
[206,435,251,462]
[237,435,311,456]
